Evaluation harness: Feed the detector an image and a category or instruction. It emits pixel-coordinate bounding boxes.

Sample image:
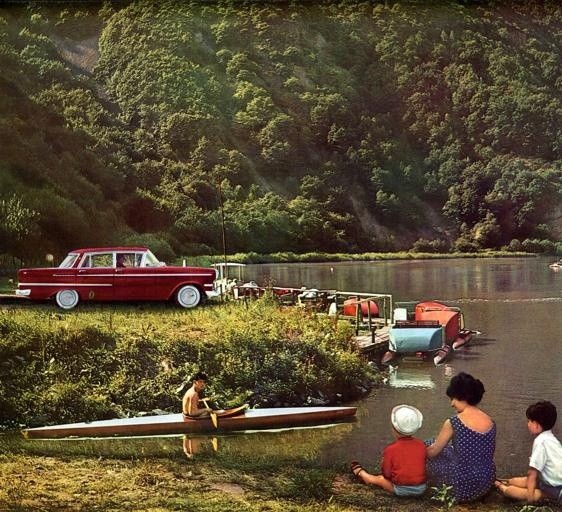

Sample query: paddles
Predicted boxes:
[210,437,218,452]
[203,399,217,428]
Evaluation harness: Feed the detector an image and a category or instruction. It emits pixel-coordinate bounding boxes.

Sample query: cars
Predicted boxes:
[15,247,219,309]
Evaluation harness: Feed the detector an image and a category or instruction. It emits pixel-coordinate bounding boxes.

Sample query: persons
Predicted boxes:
[182,372,224,418]
[226,278,238,296]
[423,372,497,503]
[350,404,428,498]
[116,253,126,268]
[182,436,208,462]
[492,399,562,506]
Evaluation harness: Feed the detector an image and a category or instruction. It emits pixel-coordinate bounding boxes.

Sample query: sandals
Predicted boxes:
[351,461,368,479]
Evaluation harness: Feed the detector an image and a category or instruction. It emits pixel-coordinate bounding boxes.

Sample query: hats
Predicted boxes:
[391,404,424,437]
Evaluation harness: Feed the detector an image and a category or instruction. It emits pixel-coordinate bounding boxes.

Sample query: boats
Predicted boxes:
[259,286,379,318]
[22,405,356,438]
[381,302,476,365]
[210,260,259,300]
